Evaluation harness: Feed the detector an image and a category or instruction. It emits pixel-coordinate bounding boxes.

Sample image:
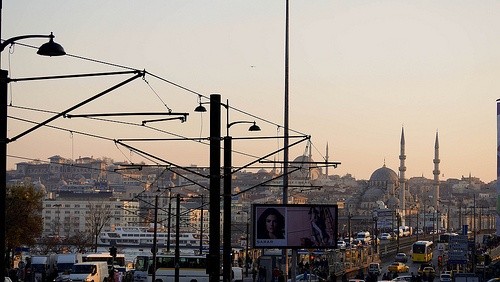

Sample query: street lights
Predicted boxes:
[397,212,401,253]
[0,30,343,282]
[372,212,378,252]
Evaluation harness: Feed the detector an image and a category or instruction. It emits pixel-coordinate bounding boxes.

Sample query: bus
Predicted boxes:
[437,232,459,251]
[131,253,224,282]
[410,240,434,263]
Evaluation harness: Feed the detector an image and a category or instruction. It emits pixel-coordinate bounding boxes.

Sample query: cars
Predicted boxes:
[439,273,452,282]
[391,275,422,282]
[338,226,441,252]
[418,266,435,277]
[394,253,408,263]
[348,278,365,282]
[387,261,410,273]
[287,273,326,282]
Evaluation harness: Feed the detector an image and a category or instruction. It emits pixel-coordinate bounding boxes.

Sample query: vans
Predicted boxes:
[367,262,382,276]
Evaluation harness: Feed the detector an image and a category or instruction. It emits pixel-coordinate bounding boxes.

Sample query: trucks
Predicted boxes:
[60,260,109,282]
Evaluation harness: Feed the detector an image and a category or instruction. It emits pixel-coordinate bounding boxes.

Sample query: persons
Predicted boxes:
[238,256,260,270]
[330,270,378,282]
[363,227,392,236]
[256,207,286,240]
[408,269,435,282]
[123,271,133,282]
[251,258,329,282]
[469,235,500,260]
[382,271,399,280]
